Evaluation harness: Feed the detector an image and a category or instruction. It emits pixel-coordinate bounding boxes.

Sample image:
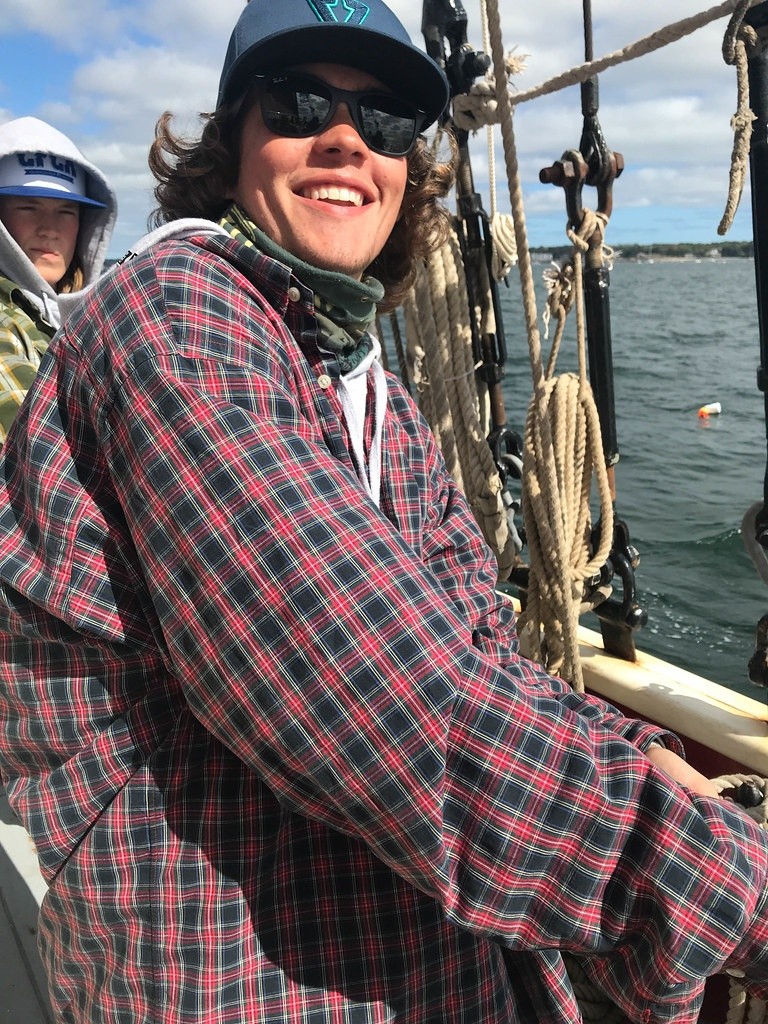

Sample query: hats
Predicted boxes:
[0,152,109,210]
[216,0,450,135]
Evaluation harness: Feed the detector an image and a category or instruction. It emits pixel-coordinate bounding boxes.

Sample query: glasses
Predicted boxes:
[235,63,431,158]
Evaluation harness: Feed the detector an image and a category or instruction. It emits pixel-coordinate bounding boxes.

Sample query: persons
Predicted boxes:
[269,113,320,134]
[0,1,768,1024]
[0,115,118,443]
[366,130,386,150]
